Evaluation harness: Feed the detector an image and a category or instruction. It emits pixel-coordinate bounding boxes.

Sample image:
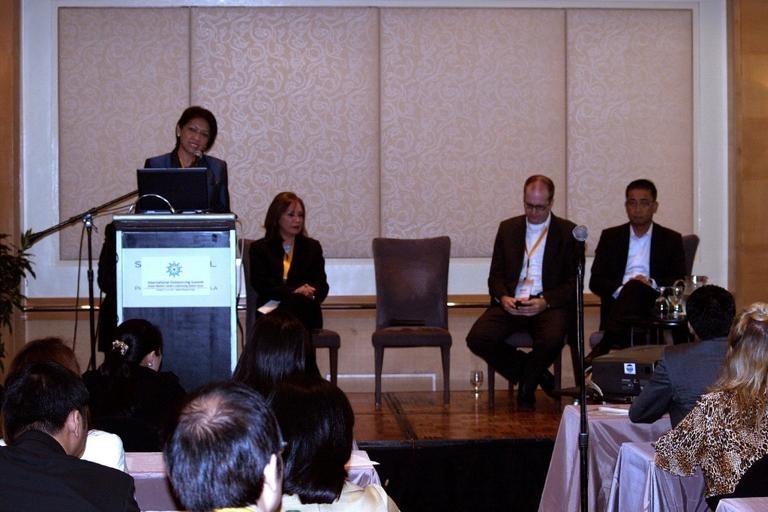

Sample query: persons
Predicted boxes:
[654,300,768,509]
[583,180,689,366]
[627,284,736,432]
[250,191,329,378]
[143,106,231,215]
[466,171,586,408]
[1,320,400,511]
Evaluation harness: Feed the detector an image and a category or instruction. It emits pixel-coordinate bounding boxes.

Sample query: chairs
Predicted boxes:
[240,238,341,390]
[372,235,451,407]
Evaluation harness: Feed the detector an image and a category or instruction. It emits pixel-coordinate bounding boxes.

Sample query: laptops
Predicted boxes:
[136,168,210,212]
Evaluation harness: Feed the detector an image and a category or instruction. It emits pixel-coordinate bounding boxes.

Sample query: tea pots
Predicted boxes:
[673,273,708,309]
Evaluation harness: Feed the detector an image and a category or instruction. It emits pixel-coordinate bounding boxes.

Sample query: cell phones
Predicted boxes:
[513,300,530,310]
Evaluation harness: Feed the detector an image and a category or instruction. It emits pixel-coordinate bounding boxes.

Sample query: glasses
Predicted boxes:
[523,199,551,211]
[627,199,654,207]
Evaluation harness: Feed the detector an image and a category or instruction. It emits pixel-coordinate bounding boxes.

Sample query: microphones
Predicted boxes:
[573,225,590,275]
[191,150,203,167]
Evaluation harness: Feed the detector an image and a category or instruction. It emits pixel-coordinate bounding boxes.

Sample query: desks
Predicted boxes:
[118,448,378,510]
[566,402,768,512]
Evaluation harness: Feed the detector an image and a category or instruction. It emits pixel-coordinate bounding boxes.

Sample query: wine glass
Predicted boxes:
[469,369,484,396]
[655,286,681,323]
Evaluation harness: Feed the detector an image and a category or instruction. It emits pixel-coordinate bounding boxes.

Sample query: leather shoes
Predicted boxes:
[537,366,560,400]
[518,382,537,409]
[560,385,602,404]
